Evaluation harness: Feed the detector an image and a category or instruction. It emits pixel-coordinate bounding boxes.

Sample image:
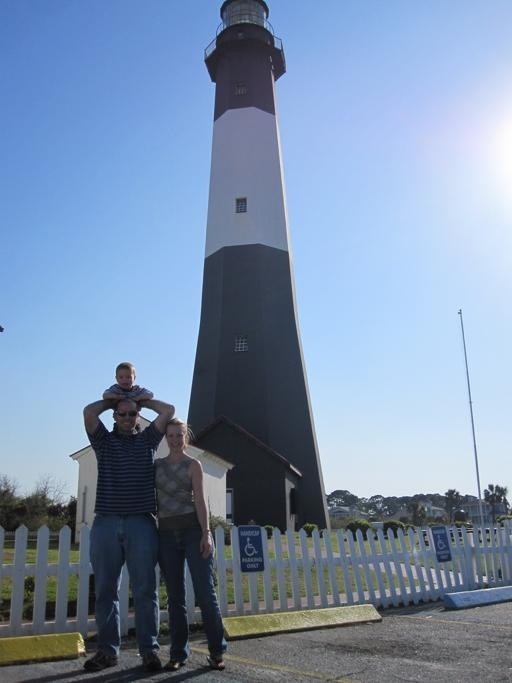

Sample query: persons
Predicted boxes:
[84,398,175,669]
[103,362,154,432]
[153,417,226,668]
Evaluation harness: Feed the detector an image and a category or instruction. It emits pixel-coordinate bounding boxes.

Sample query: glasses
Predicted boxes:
[115,409,138,418]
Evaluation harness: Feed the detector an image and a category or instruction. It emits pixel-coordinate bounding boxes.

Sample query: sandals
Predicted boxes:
[163,659,187,671]
[211,660,225,670]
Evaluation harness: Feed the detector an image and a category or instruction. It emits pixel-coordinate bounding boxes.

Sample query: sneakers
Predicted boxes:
[142,651,162,669]
[83,644,119,670]
